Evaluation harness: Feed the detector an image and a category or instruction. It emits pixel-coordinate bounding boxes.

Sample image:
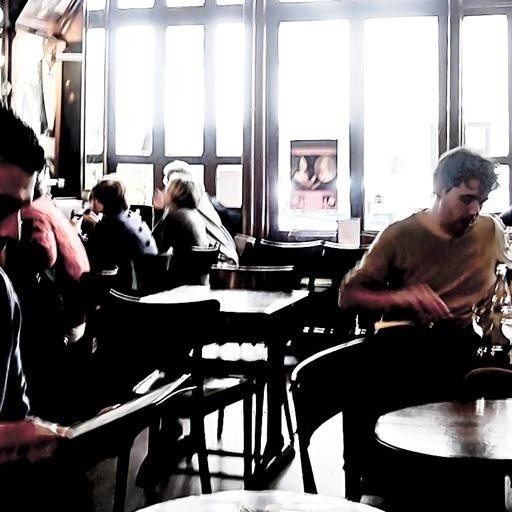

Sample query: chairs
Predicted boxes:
[0,232,512,512]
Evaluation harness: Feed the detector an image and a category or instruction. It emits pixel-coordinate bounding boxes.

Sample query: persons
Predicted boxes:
[161,157,241,268]
[336,147,506,395]
[84,180,160,331]
[293,157,322,190]
[0,105,92,509]
[151,178,210,273]
[79,190,103,237]
